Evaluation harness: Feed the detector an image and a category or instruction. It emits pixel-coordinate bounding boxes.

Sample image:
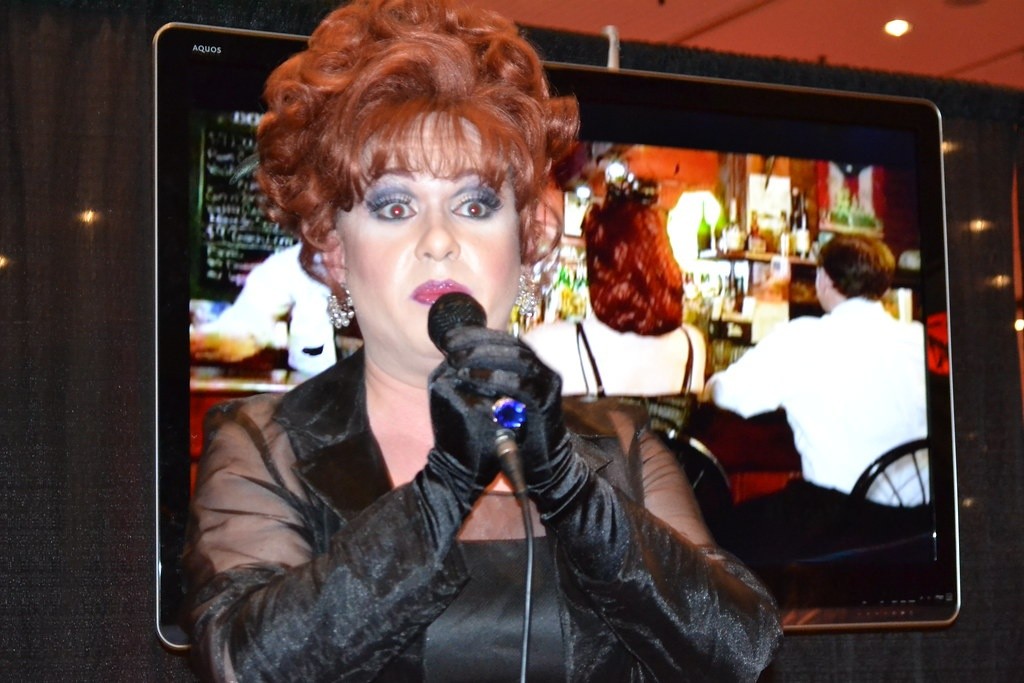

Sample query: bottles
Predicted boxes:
[698,189,875,260]
[507,264,736,343]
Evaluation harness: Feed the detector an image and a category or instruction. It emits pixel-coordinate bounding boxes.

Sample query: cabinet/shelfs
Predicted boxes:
[717,253,817,323]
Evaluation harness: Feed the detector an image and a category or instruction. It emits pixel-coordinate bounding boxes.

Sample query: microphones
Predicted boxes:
[428,291,530,499]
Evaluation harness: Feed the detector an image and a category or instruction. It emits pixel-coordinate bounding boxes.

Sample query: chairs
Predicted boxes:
[668,436,726,554]
[841,438,932,546]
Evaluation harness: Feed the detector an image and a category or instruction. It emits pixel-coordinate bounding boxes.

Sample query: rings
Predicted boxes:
[490,394,527,432]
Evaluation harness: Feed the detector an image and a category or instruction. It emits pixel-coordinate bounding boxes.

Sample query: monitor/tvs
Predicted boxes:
[149,23,964,649]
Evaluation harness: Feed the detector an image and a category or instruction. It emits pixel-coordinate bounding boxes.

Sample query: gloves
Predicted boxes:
[413,326,629,581]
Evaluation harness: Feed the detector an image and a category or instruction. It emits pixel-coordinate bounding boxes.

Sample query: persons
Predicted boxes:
[190,231,360,385]
[183,0,784,683]
[519,199,704,398]
[705,235,932,565]
